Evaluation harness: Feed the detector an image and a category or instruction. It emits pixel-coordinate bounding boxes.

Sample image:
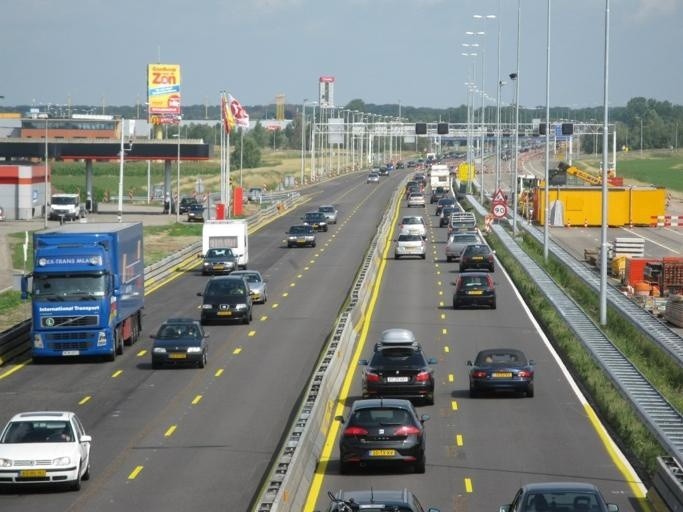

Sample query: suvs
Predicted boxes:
[355,327,439,407]
[178,196,197,215]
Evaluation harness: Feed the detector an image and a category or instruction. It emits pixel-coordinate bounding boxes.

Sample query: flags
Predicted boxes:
[221,92,249,134]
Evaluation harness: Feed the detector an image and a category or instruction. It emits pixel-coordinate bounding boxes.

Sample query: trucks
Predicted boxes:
[15,221,146,367]
[198,218,250,270]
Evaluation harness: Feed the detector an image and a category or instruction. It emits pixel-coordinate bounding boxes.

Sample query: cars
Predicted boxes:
[0,408,95,494]
[299,211,330,233]
[248,187,263,200]
[226,269,268,304]
[186,204,205,222]
[464,346,539,399]
[360,139,542,272]
[283,222,318,248]
[335,398,433,476]
[147,315,211,370]
[317,205,338,225]
[321,484,442,512]
[498,480,620,512]
[200,246,239,273]
[448,272,497,311]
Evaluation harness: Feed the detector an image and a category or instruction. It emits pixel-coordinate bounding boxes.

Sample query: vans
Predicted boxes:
[48,192,81,221]
[194,276,255,326]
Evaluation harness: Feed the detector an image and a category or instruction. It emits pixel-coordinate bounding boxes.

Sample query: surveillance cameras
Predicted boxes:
[499,81,508,86]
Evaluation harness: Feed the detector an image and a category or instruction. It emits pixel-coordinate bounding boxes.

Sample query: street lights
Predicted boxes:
[33,100,97,120]
[458,10,602,209]
[175,114,184,208]
[296,95,410,183]
